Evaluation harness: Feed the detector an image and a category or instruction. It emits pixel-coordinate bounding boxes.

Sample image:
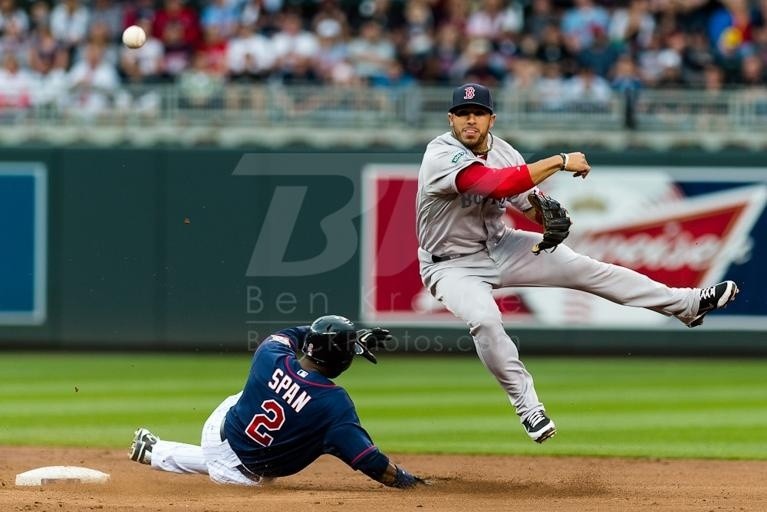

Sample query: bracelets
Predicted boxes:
[560,152,569,171]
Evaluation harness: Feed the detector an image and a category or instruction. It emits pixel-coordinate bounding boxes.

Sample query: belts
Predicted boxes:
[432,254,471,263]
[220,415,260,482]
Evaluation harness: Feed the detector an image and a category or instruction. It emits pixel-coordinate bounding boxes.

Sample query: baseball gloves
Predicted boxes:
[527,193,572,255]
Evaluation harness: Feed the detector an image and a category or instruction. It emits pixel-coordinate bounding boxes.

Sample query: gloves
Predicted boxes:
[354,327,393,364]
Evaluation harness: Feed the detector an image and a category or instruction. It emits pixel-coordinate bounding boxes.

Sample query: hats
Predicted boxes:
[448,83,493,115]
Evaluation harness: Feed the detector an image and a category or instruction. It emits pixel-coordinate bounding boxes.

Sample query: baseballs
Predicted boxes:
[123,24,147,48]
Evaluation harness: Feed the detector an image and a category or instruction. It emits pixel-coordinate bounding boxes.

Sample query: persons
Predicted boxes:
[415,82,739,444]
[0,0,767,127]
[129,315,426,489]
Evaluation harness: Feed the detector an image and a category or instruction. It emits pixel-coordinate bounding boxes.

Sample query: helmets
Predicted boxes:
[301,315,356,378]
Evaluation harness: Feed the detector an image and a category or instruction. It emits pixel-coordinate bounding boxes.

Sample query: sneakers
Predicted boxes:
[686,280,739,328]
[128,427,158,465]
[521,409,556,444]
